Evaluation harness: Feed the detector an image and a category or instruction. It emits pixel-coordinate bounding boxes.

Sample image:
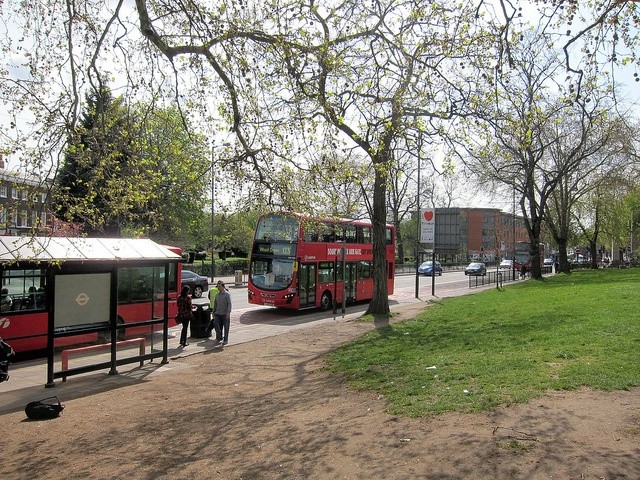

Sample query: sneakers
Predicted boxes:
[214,337,223,344]
[222,341,228,345]
[183,342,189,346]
[180,340,183,344]
[205,329,211,336]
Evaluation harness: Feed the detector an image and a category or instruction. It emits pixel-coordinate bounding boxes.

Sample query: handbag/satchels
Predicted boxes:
[174,314,182,325]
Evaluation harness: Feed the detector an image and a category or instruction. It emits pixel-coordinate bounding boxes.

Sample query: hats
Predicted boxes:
[217,280,224,285]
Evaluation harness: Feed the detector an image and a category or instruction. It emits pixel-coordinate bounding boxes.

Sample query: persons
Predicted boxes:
[214,281,232,345]
[208,280,223,319]
[177,285,196,346]
[0,288,13,311]
[520,264,527,280]
[26,286,44,309]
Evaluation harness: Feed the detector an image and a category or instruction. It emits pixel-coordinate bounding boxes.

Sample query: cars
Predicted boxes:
[180,270,209,298]
[418,260,443,277]
[544,258,554,265]
[500,260,514,269]
[465,262,487,276]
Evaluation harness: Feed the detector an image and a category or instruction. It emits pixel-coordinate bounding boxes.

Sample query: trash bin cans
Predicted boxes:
[190,303,210,338]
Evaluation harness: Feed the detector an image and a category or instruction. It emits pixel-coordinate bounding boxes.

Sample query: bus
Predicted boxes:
[0,235,183,360]
[246,211,396,313]
[513,241,545,272]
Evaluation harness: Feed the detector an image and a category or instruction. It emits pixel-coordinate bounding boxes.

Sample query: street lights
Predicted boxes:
[211,142,231,282]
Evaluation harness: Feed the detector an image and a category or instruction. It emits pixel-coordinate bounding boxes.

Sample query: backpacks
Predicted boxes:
[25,395,65,419]
[0,337,15,382]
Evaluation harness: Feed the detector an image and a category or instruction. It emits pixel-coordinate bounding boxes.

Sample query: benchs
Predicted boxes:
[60,338,146,381]
